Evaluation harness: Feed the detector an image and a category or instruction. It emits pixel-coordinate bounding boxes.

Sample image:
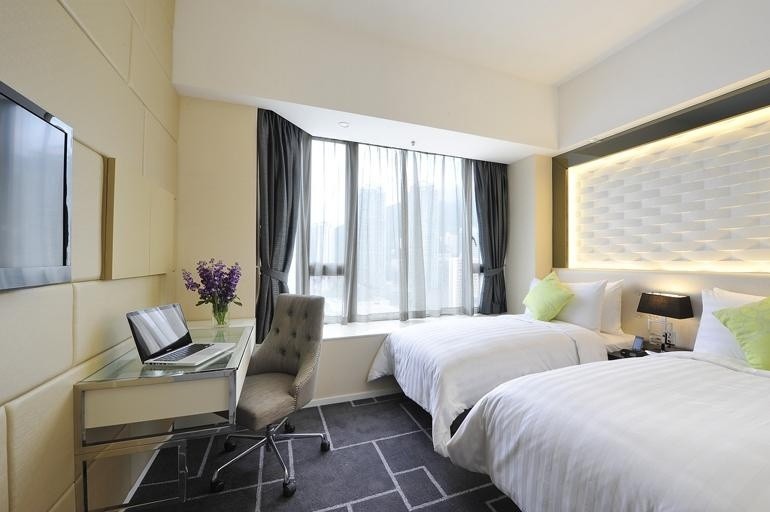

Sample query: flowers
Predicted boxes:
[181,258,244,327]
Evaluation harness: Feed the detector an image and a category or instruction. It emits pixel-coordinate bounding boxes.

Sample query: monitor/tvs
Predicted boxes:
[0,81,73,291]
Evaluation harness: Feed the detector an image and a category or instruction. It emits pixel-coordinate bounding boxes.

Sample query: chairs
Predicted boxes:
[210,292,330,497]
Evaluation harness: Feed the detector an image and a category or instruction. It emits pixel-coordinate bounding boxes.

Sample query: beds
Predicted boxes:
[365,266,644,457]
[448,286,769,510]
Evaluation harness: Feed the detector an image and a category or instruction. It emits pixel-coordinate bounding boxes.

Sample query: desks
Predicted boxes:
[73,325,257,511]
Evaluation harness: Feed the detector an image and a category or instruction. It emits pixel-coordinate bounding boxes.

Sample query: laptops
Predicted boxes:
[125,303,237,367]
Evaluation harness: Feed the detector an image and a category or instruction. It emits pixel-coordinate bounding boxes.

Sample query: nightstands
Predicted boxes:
[609,343,692,360]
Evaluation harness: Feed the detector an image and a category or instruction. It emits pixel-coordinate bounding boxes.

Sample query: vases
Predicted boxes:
[211,300,229,325]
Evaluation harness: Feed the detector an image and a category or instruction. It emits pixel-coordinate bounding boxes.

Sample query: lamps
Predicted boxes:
[637,293,695,350]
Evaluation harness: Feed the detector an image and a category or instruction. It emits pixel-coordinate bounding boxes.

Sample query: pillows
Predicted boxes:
[708,296,770,368]
[604,277,626,336]
[524,271,575,321]
[712,287,763,304]
[689,290,744,362]
[523,277,608,334]
